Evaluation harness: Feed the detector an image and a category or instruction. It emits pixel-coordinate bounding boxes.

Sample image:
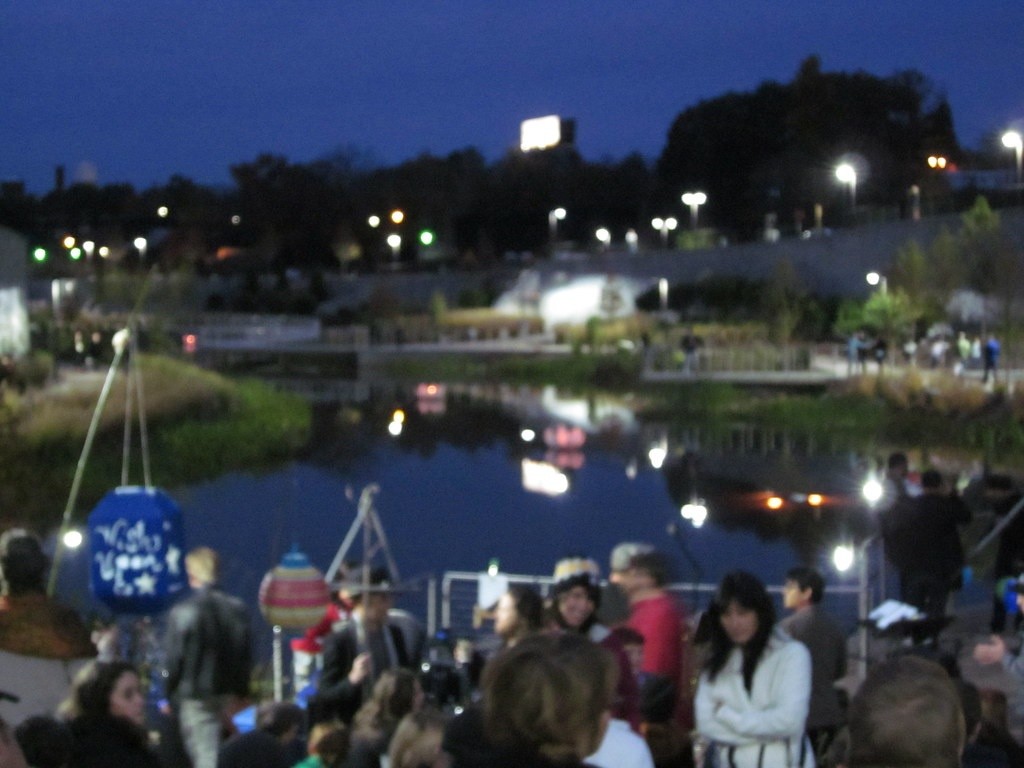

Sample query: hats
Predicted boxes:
[611,545,664,578]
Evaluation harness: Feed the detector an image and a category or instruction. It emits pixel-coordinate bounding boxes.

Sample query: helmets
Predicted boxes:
[550,557,602,611]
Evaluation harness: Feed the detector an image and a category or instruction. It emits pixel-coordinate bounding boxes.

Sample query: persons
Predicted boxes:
[880,453,968,644]
[846,327,1001,385]
[0,529,1024,768]
